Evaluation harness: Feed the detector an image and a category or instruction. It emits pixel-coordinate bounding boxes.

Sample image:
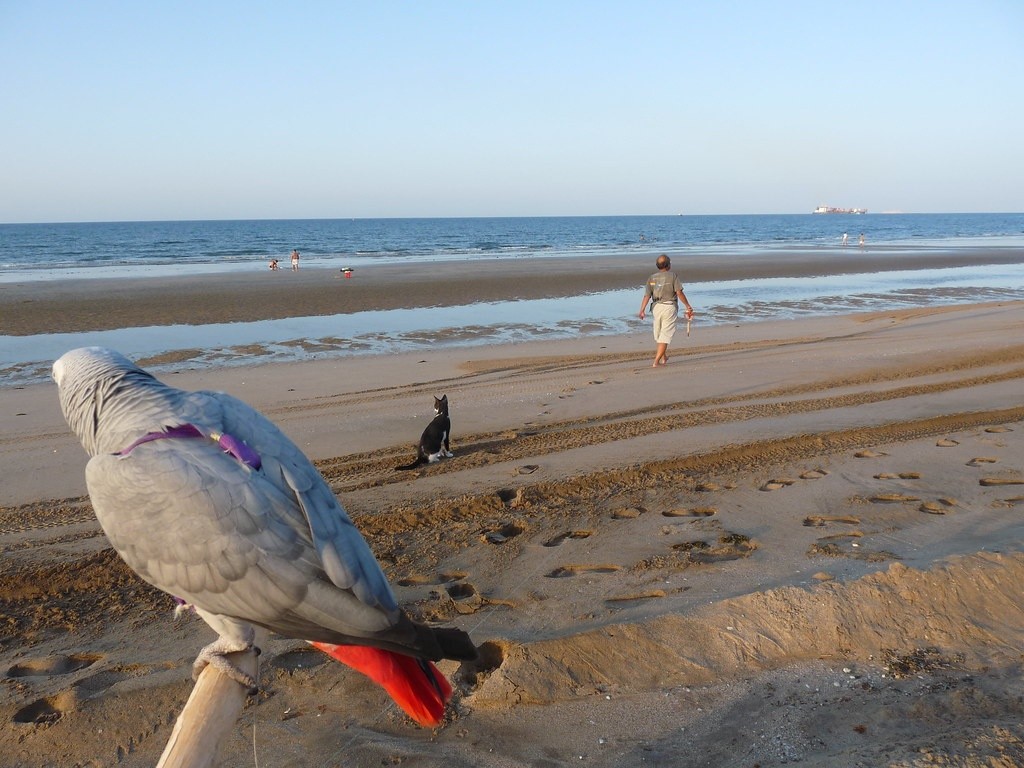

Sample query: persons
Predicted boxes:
[640,234,644,241]
[268,259,279,271]
[290,249,300,272]
[654,237,658,241]
[638,254,695,368]
[841,232,849,246]
[859,233,865,248]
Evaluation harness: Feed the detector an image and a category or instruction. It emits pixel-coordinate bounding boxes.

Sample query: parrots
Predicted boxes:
[51,346,480,728]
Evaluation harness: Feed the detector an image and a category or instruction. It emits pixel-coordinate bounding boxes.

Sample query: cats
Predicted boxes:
[393,394,454,471]
